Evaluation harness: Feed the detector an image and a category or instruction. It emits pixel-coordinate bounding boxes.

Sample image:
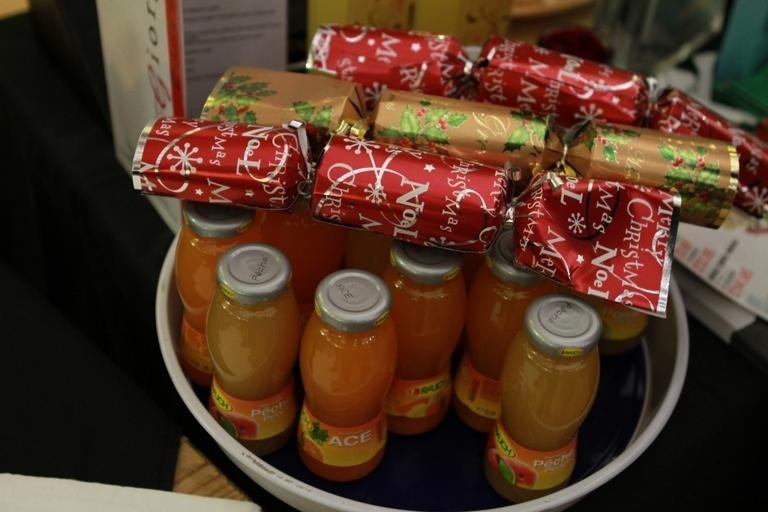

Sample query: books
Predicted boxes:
[667,257,768,380]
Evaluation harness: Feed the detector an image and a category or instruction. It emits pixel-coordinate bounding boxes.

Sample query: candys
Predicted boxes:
[129,21,768,320]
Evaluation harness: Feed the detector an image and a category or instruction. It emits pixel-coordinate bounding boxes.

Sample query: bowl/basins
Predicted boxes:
[153,207,690,512]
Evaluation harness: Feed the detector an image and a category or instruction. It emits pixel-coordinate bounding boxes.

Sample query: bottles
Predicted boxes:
[204,245,301,455]
[174,200,641,391]
[451,231,555,435]
[379,239,469,438]
[485,298,604,501]
[296,271,399,484]
